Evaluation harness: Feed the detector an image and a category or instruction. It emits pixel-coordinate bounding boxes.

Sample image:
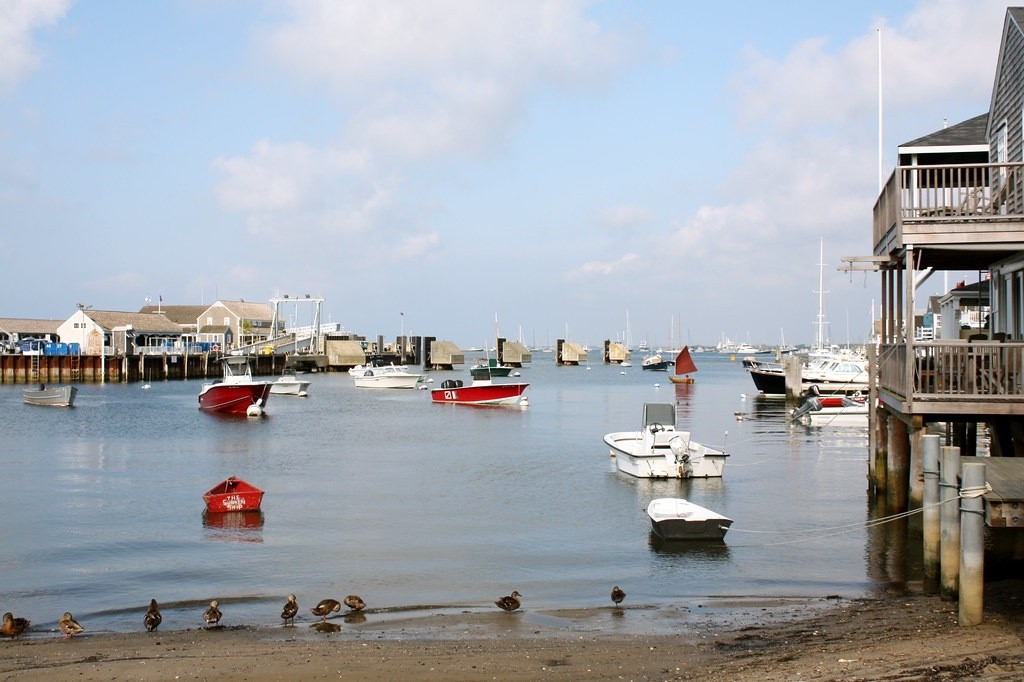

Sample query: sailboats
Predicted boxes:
[668,345,698,384]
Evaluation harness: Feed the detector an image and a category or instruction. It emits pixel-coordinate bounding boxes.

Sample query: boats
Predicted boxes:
[717,236,884,419]
[200,474,265,515]
[459,312,696,354]
[602,401,730,479]
[643,498,734,542]
[470,359,514,377]
[269,369,311,397]
[23,383,78,407]
[431,371,530,405]
[348,361,422,388]
[198,350,274,416]
[641,348,667,371]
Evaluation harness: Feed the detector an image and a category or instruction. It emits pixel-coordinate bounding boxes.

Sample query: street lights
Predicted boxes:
[76,303,93,355]
[400,312,403,365]
[240,298,248,356]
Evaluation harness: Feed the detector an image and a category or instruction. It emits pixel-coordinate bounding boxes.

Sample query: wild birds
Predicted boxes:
[494,591,521,612]
[57,612,84,638]
[143,598,162,633]
[611,586,626,605]
[311,598,341,619]
[345,595,366,612]
[0,613,31,638]
[281,595,299,622]
[203,601,222,629]
